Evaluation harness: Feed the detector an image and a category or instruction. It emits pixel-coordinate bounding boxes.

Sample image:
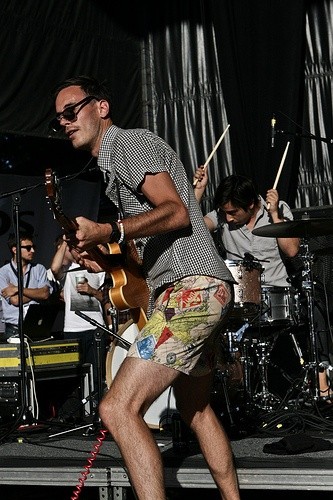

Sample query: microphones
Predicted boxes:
[271,114,276,148]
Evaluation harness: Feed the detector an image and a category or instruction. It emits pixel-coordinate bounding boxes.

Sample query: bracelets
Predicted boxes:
[117,219,124,244]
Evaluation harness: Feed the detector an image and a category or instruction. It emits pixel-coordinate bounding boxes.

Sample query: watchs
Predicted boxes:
[107,221,120,244]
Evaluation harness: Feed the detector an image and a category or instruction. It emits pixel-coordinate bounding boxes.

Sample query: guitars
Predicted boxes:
[43,167,148,310]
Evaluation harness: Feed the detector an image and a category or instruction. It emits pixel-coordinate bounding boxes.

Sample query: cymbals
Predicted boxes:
[248,217,331,240]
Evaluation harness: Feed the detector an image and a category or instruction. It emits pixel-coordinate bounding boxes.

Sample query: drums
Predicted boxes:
[103,313,182,436]
[256,282,299,332]
[222,256,264,318]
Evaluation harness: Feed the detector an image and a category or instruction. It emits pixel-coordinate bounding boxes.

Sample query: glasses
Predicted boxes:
[21,245,35,252]
[54,94,100,121]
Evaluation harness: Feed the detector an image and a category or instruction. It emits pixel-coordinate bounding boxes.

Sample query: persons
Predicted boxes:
[0,232,52,342]
[52,72,241,500]
[46,232,112,367]
[191,165,333,405]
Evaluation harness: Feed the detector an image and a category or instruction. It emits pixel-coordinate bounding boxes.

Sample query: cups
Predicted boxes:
[75,273,85,284]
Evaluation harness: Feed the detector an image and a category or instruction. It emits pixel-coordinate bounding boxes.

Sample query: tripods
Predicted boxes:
[225,238,333,418]
[47,333,110,439]
